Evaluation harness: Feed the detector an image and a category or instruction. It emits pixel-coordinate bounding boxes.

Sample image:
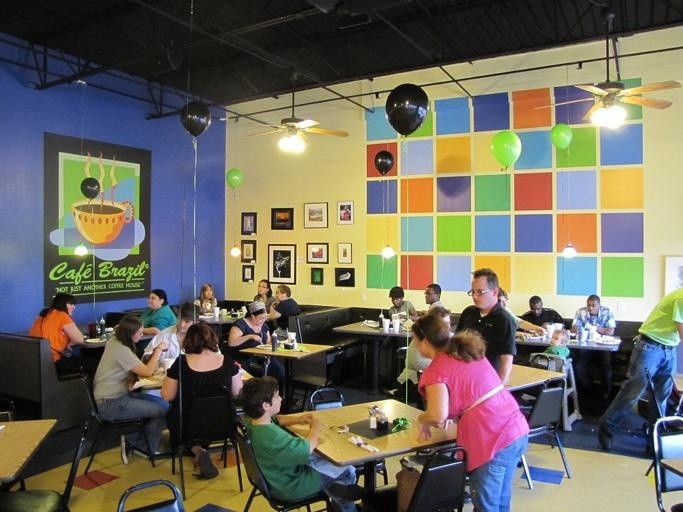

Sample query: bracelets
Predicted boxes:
[153,347,162,352]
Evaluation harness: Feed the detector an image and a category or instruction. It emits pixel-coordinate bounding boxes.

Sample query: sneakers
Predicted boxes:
[131,445,161,460]
[196,449,218,479]
[329,482,367,501]
[120,434,132,464]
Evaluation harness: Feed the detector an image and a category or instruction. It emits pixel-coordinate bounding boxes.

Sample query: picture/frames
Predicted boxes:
[240,199,355,288]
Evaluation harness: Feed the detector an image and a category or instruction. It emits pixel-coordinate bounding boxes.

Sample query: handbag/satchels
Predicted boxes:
[396,469,420,512]
[55,359,81,381]
[244,356,267,378]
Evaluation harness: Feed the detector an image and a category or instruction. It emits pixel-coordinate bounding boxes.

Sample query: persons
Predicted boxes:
[27,292,84,376]
[92,314,170,464]
[412,314,529,511]
[135,289,177,360]
[453,268,516,503]
[598,290,682,457]
[142,301,200,455]
[161,323,243,479]
[228,281,299,415]
[342,206,352,220]
[397,306,455,411]
[498,287,572,375]
[571,295,615,424]
[194,283,217,316]
[367,284,446,394]
[241,376,357,512]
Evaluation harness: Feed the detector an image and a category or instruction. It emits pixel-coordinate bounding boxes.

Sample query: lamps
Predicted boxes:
[278,128,307,158]
[380,93,396,261]
[74,80,90,257]
[590,98,629,134]
[230,117,242,259]
[561,65,577,260]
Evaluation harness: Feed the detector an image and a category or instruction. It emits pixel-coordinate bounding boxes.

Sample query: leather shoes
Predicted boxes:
[598,428,613,450]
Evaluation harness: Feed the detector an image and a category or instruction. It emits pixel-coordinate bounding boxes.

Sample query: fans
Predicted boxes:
[241,71,349,154]
[533,13,682,121]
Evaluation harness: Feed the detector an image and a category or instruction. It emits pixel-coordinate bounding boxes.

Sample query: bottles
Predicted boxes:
[271,331,278,351]
[379,312,384,328]
[99,317,106,337]
[369,409,377,429]
[89,323,96,339]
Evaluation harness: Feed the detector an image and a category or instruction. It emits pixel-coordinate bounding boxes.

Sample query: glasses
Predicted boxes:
[467,288,494,297]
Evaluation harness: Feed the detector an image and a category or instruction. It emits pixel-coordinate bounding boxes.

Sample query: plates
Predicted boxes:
[256,345,272,349]
[85,338,106,343]
[593,338,622,345]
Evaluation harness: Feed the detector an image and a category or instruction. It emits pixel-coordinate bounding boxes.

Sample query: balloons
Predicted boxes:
[384,82,430,136]
[548,122,574,154]
[79,176,101,202]
[224,168,246,194]
[374,149,394,177]
[178,98,213,139]
[488,129,522,170]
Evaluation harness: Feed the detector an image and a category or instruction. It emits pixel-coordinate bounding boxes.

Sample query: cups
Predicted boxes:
[546,323,596,341]
[392,314,399,320]
[392,320,400,333]
[287,332,296,343]
[382,319,390,333]
[214,307,220,317]
[220,309,227,317]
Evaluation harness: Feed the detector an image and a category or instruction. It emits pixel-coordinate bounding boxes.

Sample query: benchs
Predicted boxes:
[0,301,683,434]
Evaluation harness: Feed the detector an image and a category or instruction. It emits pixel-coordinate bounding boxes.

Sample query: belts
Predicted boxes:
[641,333,672,350]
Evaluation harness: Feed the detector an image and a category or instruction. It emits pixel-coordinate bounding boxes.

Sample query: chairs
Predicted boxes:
[0,347,683,512]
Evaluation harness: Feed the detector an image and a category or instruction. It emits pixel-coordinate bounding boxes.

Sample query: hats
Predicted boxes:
[248,302,266,314]
[173,302,205,321]
